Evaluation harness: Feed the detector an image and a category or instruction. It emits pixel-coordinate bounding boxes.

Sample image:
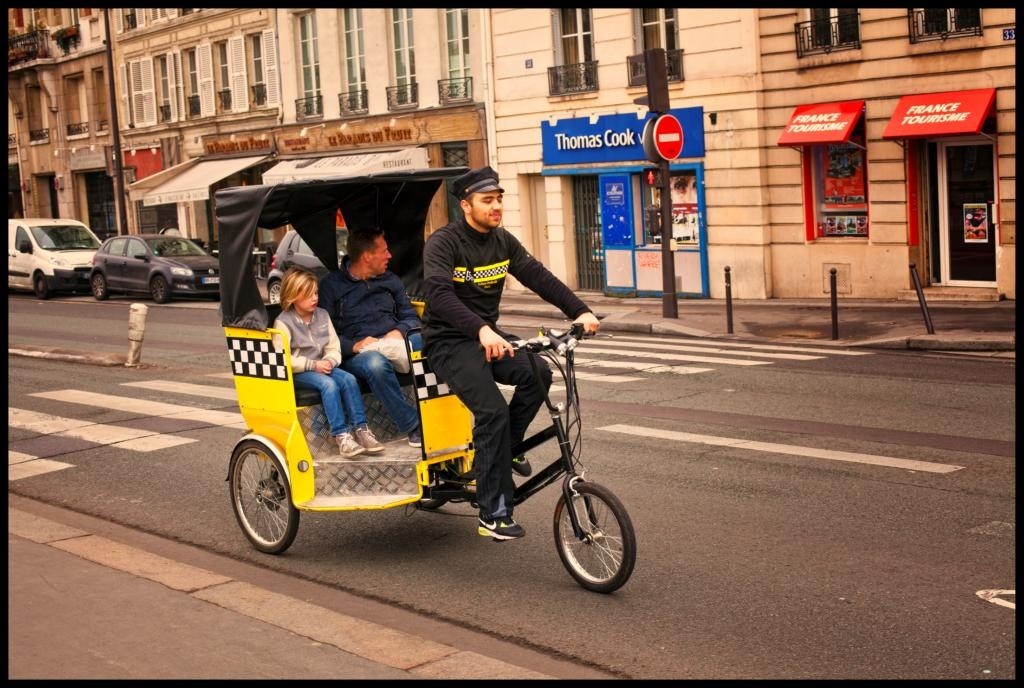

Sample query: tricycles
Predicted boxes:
[215,167,638,594]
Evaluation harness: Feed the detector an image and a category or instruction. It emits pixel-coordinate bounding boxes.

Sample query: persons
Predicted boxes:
[318,226,424,447]
[274,267,386,457]
[422,167,599,540]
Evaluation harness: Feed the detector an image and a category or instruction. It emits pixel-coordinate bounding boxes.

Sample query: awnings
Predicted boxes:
[144,154,268,207]
[777,99,866,149]
[261,147,431,185]
[882,88,997,140]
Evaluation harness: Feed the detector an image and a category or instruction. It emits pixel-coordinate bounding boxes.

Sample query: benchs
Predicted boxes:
[265,302,411,457]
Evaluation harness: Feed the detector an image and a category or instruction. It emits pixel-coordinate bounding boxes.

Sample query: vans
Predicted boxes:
[7,218,103,300]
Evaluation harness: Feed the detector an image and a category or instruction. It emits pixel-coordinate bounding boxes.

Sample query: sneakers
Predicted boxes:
[478,516,525,541]
[511,453,532,476]
[354,424,385,452]
[336,432,365,457]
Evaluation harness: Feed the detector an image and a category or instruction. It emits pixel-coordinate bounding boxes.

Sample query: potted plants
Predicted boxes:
[8,22,78,65]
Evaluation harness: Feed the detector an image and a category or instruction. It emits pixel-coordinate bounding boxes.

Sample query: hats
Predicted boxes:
[450,166,505,200]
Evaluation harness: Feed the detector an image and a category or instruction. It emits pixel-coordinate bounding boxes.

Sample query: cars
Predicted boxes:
[89,234,221,304]
[267,227,348,305]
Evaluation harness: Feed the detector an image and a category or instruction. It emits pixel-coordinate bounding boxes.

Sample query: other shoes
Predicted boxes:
[408,425,422,447]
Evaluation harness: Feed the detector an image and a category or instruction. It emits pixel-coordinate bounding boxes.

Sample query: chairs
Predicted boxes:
[192,238,278,279]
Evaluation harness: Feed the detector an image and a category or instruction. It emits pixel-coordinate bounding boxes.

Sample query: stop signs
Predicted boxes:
[653,114,683,162]
